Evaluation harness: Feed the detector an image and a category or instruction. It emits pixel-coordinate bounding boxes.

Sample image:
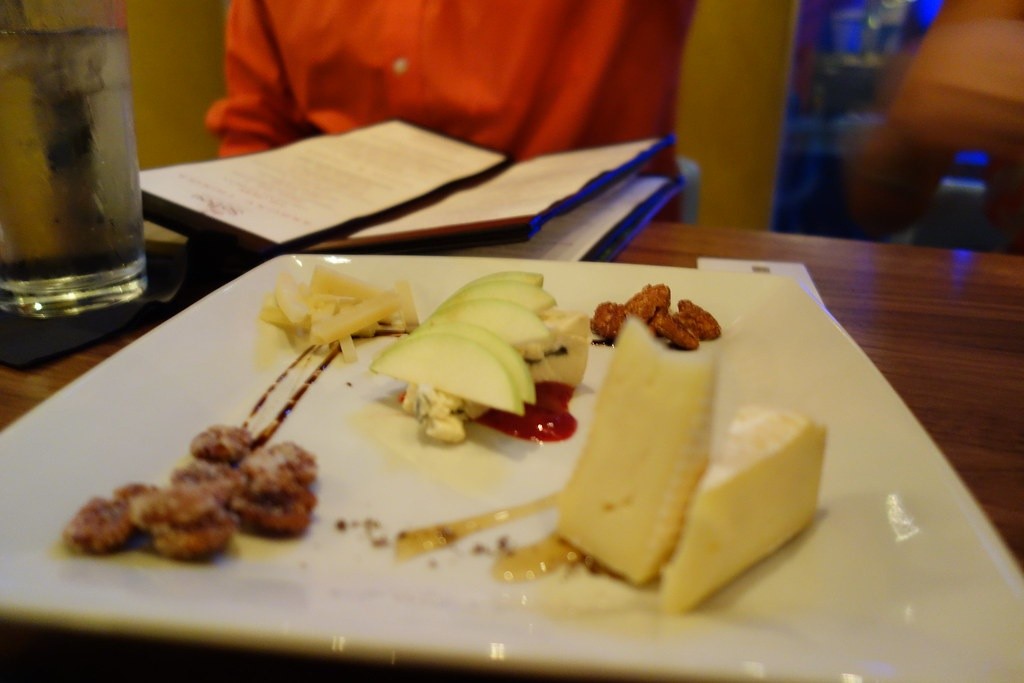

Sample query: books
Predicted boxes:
[139,117,681,263]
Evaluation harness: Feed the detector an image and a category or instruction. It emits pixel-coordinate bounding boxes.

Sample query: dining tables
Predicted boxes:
[0,220,1024,683]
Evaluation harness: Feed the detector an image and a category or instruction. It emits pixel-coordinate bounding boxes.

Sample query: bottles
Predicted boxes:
[911,149,1005,252]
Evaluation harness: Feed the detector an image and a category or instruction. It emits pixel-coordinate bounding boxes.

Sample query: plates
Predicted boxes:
[0,253,1024,683]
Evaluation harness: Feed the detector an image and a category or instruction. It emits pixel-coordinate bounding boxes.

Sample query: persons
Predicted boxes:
[204,0,701,221]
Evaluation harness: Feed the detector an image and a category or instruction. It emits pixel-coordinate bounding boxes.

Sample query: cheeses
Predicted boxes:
[556,318,829,615]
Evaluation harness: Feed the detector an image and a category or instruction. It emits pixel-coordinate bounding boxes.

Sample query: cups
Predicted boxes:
[0,0,147,319]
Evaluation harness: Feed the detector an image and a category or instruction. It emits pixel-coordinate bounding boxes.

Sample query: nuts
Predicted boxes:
[589,284,721,351]
[63,420,327,565]
